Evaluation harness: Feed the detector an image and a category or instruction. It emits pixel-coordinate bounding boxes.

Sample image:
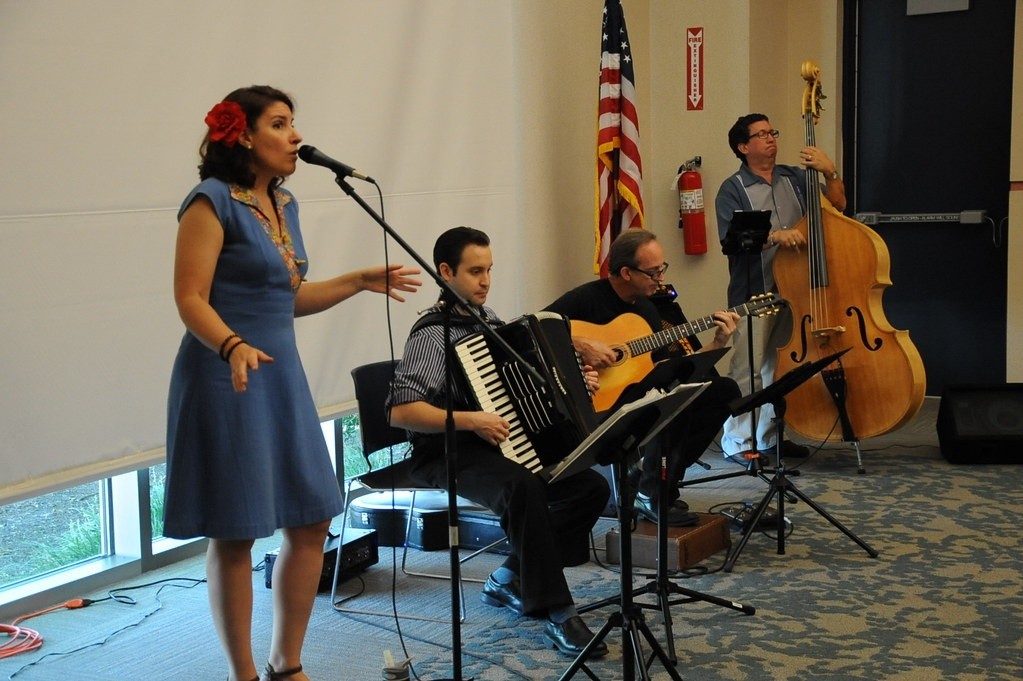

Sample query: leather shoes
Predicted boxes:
[480,570,525,613]
[633,493,700,525]
[760,441,810,459]
[542,614,609,656]
[724,451,768,466]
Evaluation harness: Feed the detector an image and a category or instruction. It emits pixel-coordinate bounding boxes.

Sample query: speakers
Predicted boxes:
[937,383,1023,465]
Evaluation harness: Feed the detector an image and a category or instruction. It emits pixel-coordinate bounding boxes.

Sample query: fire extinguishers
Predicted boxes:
[676,157,708,255]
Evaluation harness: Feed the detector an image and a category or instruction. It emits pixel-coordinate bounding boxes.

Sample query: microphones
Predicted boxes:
[298,145,376,184]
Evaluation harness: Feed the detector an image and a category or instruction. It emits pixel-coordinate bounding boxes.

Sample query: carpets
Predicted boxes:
[409,456,1023,681]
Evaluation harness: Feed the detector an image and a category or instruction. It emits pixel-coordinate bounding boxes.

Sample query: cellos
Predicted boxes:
[768,56,926,474]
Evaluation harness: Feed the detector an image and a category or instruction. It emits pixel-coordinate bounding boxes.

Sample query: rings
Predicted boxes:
[792,241,796,248]
[809,156,811,160]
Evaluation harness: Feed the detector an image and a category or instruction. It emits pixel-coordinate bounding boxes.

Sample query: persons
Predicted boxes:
[384,227,611,656]
[541,228,741,527]
[713,114,847,465]
[162,85,423,680]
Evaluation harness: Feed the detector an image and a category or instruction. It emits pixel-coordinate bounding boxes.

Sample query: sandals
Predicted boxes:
[260,663,302,681]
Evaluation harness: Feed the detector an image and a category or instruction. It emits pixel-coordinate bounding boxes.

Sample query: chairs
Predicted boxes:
[330,359,507,626]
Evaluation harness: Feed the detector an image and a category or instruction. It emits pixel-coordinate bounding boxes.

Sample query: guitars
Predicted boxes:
[569,290,782,413]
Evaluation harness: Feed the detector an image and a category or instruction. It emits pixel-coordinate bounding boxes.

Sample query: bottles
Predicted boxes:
[379,661,411,681]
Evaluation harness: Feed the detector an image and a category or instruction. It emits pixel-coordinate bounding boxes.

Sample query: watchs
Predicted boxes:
[823,170,839,180]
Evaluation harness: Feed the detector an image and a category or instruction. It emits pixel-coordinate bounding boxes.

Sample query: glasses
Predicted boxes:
[617,262,669,281]
[743,128,780,145]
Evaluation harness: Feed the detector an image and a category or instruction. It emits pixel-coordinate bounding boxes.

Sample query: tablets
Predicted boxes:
[721,209,773,255]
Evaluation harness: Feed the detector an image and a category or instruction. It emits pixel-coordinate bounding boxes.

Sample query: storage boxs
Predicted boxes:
[605,511,730,573]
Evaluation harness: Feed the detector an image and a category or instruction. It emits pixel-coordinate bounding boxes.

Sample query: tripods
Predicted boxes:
[548,228,882,681]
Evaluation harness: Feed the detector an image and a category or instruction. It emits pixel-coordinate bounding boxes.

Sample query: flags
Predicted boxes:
[593,0,646,278]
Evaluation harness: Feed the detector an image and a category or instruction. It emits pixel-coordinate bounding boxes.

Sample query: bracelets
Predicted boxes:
[219,334,240,361]
[769,231,776,247]
[226,340,248,364]
